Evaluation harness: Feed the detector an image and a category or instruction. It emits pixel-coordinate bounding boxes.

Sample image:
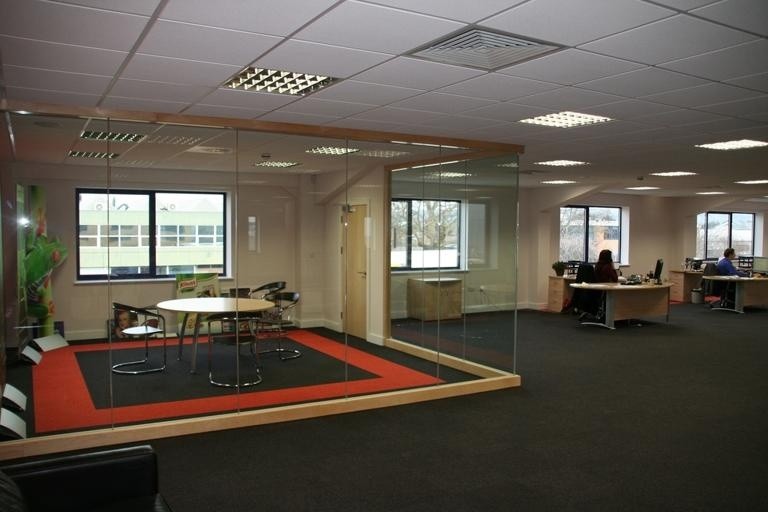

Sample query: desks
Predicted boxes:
[568,279,675,330]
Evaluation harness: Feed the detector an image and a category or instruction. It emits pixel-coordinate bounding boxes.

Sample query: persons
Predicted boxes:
[115,311,133,338]
[716,247,753,277]
[594,249,619,283]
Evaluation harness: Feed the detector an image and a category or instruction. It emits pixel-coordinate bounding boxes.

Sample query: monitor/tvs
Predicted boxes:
[753,257,768,276]
[653,259,663,285]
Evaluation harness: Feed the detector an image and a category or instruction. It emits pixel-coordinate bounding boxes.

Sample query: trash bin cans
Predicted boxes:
[691,288,704,304]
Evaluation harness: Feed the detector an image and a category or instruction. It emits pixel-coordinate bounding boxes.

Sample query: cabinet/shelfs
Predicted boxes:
[547,275,572,313]
[701,275,768,314]
[407,278,465,322]
[668,268,704,303]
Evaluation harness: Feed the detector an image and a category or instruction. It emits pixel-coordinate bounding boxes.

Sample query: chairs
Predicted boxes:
[109,280,303,389]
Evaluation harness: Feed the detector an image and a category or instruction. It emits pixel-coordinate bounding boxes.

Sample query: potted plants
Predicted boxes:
[551,261,568,276]
